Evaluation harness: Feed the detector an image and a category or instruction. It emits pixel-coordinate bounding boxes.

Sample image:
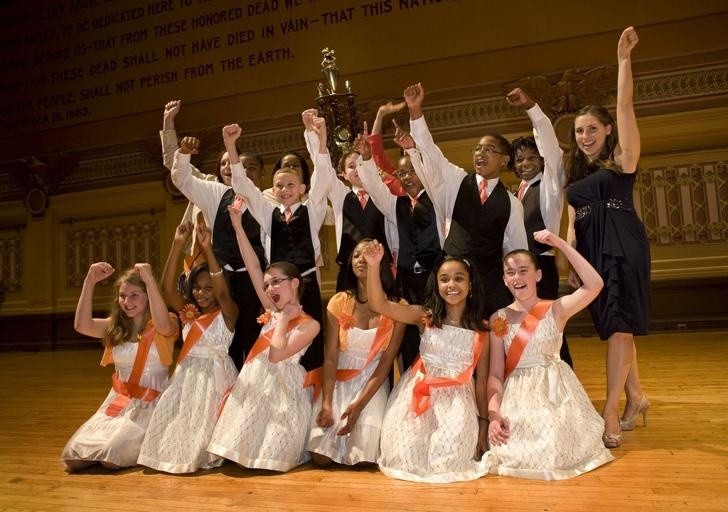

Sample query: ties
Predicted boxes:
[412,196,416,212]
[357,190,367,209]
[479,179,487,204]
[517,180,528,201]
[284,208,291,224]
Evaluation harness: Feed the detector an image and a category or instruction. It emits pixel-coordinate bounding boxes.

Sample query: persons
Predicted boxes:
[60,26,651,482]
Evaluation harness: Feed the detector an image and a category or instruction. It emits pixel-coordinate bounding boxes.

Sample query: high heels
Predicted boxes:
[620,395,649,431]
[601,416,623,448]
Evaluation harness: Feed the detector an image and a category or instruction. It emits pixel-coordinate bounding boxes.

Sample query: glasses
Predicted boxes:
[262,278,288,291]
[471,145,504,157]
